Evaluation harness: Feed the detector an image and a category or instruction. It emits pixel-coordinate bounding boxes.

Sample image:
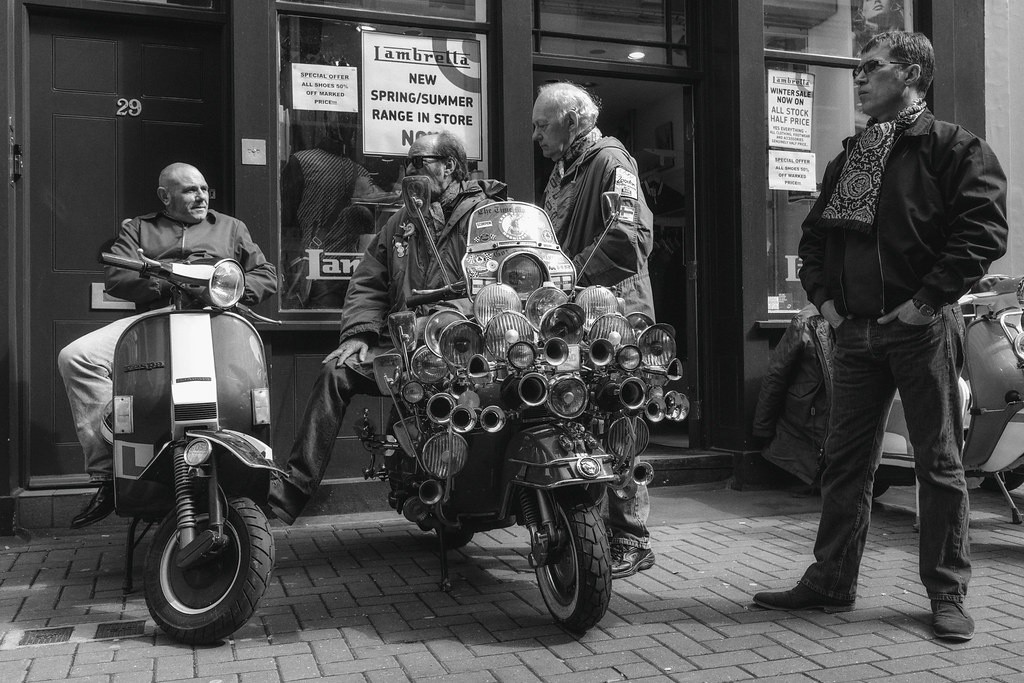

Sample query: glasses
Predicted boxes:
[852,59,912,79]
[403,155,448,169]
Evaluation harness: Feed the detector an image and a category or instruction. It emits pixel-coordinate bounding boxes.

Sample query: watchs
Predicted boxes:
[912,299,935,317]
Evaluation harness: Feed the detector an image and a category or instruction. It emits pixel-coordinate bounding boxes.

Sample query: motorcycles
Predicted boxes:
[353,175,694,632]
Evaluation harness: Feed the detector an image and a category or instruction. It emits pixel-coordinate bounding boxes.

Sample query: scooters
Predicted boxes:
[812,273,1023,526]
[97,249,290,645]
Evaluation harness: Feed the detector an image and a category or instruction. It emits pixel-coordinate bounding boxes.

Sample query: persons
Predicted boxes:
[267,133,508,526]
[57,162,278,529]
[532,83,653,578]
[280,137,376,310]
[752,31,1008,640]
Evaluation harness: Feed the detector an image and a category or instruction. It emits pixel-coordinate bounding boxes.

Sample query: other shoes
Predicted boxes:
[930,599,976,640]
[752,581,857,613]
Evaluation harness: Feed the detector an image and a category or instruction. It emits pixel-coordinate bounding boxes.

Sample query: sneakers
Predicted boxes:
[268,478,311,525]
[610,542,655,580]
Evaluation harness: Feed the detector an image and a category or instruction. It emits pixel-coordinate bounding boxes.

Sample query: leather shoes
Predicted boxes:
[71,484,114,529]
